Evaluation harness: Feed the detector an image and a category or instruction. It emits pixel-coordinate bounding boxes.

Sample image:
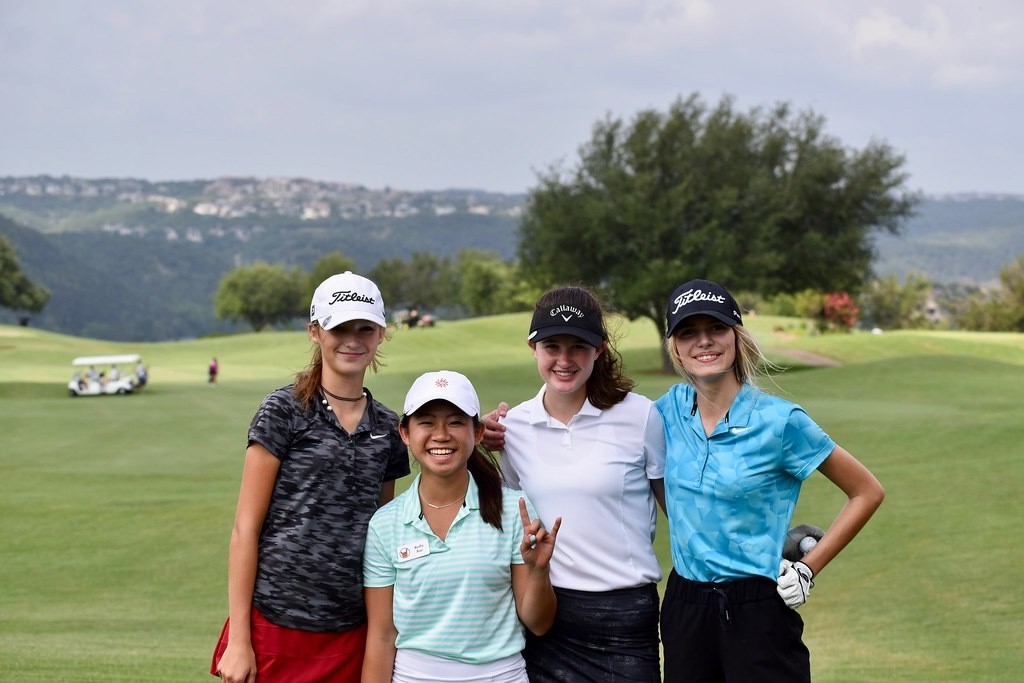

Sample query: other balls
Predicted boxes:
[799,536,818,554]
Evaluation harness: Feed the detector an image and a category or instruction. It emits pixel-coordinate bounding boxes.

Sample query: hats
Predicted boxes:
[664,278,744,341]
[526,303,610,350]
[401,369,482,423]
[308,270,387,332]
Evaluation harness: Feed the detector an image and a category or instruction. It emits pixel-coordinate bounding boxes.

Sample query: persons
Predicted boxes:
[110,364,119,381]
[209,271,410,683]
[137,361,146,393]
[479,278,886,683]
[209,357,218,385]
[88,365,97,380]
[361,369,562,683]
[496,286,825,683]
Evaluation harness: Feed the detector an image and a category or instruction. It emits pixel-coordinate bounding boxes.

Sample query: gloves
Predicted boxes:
[776,558,816,611]
[782,524,826,563]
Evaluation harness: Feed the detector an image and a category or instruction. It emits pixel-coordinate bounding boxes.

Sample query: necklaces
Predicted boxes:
[322,386,367,411]
[419,491,465,508]
